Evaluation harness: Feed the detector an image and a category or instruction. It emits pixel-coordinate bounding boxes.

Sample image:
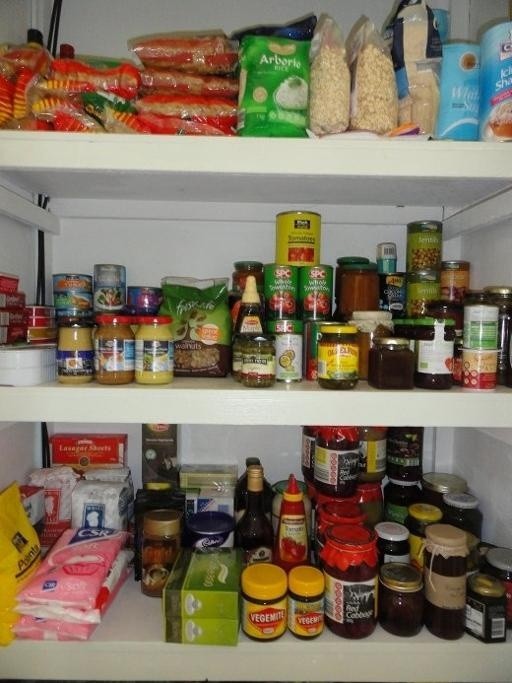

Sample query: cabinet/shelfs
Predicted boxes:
[1,125,511,680]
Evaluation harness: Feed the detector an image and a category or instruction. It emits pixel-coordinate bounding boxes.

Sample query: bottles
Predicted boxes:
[232,456,310,575]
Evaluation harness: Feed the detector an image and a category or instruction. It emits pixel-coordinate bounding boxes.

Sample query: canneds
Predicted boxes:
[230,212,512,389]
[136,507,236,598]
[54,263,174,385]
[302,426,512,643]
[241,564,325,640]
